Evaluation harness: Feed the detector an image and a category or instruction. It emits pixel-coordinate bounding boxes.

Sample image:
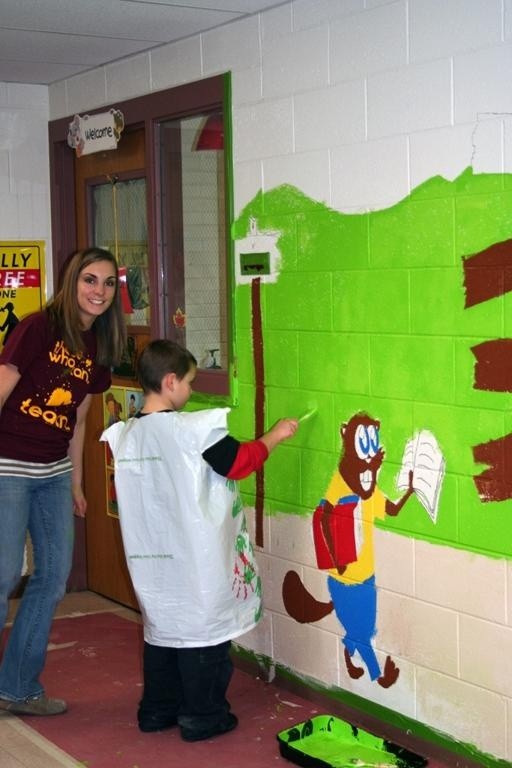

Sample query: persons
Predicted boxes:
[104,392,122,427]
[0,247,129,718]
[0,302,20,347]
[108,474,118,514]
[98,338,300,744]
[128,395,137,418]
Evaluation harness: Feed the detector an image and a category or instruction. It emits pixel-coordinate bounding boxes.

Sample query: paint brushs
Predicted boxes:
[298,409,318,427]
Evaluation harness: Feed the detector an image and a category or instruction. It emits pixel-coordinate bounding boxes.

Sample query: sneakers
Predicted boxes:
[138,716,178,730]
[183,711,238,741]
[0,692,67,716]
